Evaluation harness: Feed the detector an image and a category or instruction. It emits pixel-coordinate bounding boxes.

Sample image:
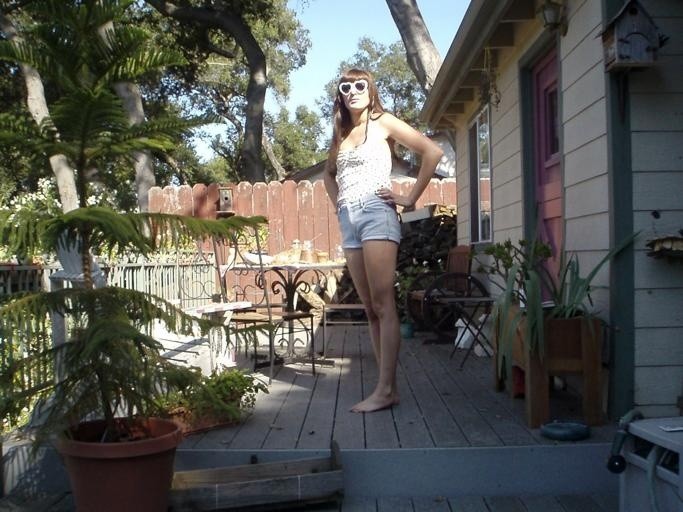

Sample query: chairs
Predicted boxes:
[405,238,472,341]
[212,223,320,385]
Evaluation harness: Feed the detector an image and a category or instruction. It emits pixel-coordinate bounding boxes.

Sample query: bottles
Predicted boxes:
[288,238,328,265]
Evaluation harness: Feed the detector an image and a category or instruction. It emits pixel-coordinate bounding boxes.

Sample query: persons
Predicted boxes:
[323,69,443,414]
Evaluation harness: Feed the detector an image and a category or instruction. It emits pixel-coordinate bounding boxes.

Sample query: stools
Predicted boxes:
[317,300,367,358]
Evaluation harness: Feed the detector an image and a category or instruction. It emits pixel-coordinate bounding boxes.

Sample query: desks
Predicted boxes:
[430,293,496,369]
[224,255,352,369]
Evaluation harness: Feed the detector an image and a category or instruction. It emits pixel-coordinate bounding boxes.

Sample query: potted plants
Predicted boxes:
[141,360,270,437]
[487,197,648,429]
[0,0,265,512]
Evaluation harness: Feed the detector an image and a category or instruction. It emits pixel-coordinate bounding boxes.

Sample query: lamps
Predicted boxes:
[535,0,567,35]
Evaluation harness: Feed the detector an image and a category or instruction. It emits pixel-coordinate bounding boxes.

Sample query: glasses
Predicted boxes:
[339,80,368,96]
